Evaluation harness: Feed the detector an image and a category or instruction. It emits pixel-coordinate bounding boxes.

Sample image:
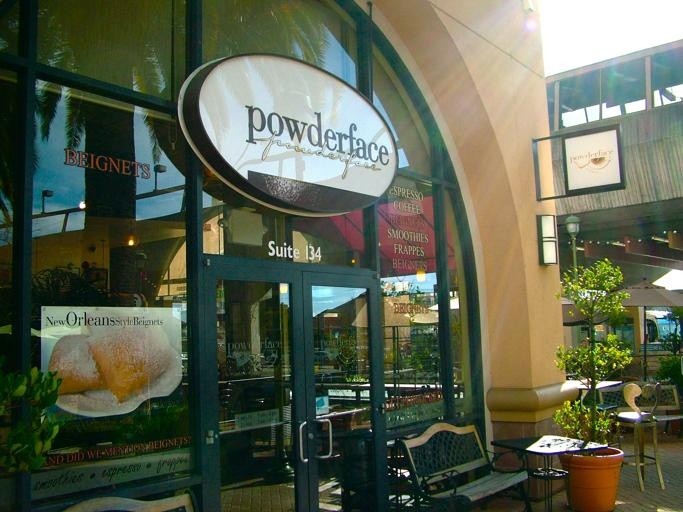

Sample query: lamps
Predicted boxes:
[536,213,558,268]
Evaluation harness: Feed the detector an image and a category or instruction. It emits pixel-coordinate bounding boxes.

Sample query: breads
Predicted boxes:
[48,334,108,394]
[87,327,170,403]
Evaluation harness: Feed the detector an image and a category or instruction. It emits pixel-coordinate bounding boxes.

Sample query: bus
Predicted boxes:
[603,308,680,349]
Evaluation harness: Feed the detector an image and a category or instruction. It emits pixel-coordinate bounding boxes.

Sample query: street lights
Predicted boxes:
[564,214,582,309]
[132,251,147,293]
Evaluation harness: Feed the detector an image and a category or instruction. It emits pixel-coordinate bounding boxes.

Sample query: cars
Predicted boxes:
[32,374,189,442]
[173,344,415,381]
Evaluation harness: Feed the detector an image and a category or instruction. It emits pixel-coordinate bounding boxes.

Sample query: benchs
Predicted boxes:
[591,383,683,438]
[55,487,200,512]
[389,415,537,511]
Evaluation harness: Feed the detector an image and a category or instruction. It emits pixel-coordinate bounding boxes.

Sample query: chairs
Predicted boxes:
[610,379,668,495]
[568,385,624,451]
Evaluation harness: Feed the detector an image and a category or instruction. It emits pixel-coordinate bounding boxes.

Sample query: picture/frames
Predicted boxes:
[54,266,80,294]
[83,267,109,300]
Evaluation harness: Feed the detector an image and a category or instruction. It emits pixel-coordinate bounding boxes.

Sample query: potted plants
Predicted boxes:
[541,252,641,511]
[650,353,682,438]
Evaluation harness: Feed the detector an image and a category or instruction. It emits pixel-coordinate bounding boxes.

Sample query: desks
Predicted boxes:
[573,377,625,406]
[484,431,611,510]
